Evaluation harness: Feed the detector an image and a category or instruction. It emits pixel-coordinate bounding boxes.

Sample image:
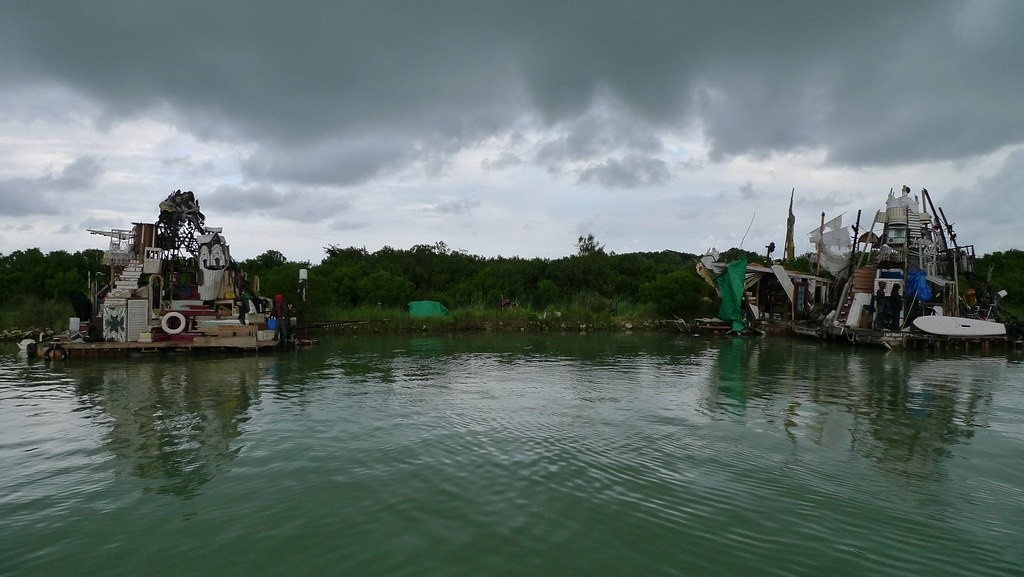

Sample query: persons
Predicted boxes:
[889,284,903,333]
[270,295,289,341]
[875,281,888,330]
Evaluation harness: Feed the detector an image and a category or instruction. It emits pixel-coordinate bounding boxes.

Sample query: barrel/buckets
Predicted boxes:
[268,319,278,329]
[932,306,944,316]
[256,331,264,341]
[69,317,80,332]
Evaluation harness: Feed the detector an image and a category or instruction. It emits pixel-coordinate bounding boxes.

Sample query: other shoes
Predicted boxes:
[272,337,277,340]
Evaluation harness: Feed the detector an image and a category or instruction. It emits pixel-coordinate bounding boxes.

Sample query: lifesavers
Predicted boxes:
[160,311,187,335]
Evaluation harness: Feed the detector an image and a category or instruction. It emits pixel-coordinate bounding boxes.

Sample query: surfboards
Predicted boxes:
[913,315,1007,336]
